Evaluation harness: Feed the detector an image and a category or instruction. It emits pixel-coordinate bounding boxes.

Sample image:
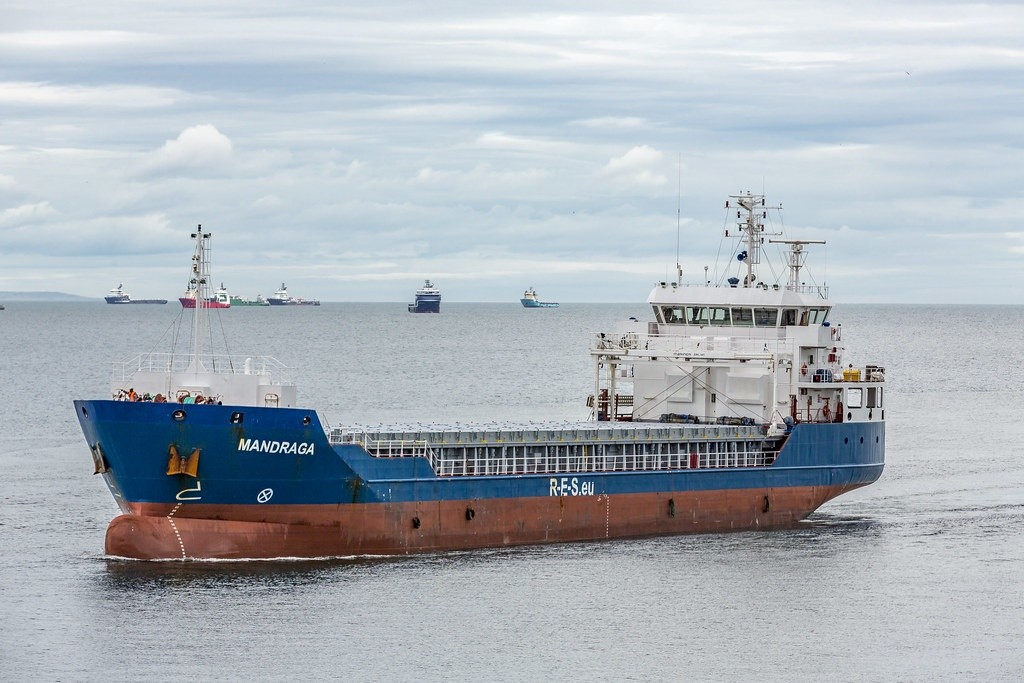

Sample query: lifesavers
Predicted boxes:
[802,365,808,374]
[466,507,476,519]
[413,517,421,528]
[823,406,828,416]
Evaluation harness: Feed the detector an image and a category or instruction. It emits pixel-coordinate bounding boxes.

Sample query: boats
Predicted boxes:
[179,281,230,308]
[71,185,889,566]
[267,281,321,307]
[519,288,560,309]
[205,292,270,306]
[406,279,442,313]
[103,283,168,305]
